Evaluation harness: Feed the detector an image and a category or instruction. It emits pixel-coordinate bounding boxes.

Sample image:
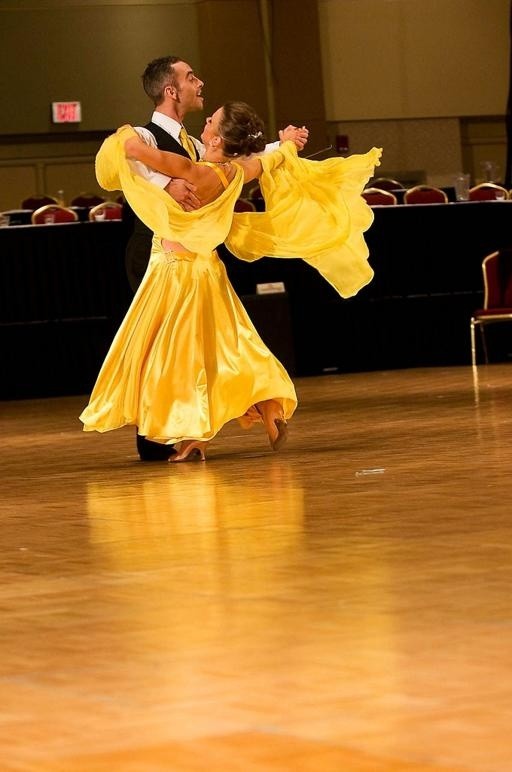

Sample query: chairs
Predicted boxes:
[88,201,123,222]
[29,203,79,225]
[234,174,512,212]
[467,242,512,394]
[20,193,58,211]
[70,191,105,208]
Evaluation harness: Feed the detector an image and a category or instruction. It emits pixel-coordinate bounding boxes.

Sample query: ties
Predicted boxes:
[180,128,197,161]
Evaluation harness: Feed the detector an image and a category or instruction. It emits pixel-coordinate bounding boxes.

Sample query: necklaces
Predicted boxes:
[203,160,232,167]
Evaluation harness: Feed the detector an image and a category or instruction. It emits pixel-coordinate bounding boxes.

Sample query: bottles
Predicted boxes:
[56,190,66,209]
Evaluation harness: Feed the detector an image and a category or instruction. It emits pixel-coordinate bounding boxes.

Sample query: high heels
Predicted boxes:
[168,440,208,462]
[265,402,289,452]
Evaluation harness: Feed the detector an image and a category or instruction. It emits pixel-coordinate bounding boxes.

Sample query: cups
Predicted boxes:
[1,216,10,227]
[44,214,55,223]
[452,174,470,201]
[94,210,107,220]
[494,192,505,201]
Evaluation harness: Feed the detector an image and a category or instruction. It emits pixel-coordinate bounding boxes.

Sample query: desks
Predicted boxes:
[3,206,92,225]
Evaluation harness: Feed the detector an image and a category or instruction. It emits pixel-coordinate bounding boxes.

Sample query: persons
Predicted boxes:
[116,55,311,462]
[93,102,310,461]
